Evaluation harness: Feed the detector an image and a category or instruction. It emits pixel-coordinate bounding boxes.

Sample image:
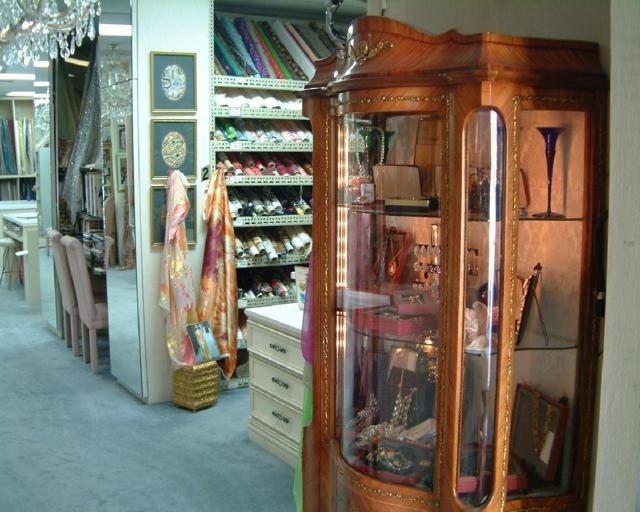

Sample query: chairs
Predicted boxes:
[60,236,108,373]
[46,231,80,358]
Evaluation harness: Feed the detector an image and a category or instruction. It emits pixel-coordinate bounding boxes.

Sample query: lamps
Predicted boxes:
[99,23,132,36]
[0,0,98,68]
[100,43,131,120]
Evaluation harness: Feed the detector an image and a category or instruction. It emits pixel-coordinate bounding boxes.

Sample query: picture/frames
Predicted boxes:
[116,125,126,154]
[150,119,197,182]
[150,51,197,116]
[150,186,197,246]
[115,154,127,192]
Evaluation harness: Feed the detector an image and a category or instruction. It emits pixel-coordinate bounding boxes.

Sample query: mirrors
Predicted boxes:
[97,0,142,396]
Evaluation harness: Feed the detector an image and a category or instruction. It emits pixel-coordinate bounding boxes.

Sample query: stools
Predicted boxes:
[0,237,22,290]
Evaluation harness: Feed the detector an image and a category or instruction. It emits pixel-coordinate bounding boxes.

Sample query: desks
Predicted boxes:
[2,213,40,304]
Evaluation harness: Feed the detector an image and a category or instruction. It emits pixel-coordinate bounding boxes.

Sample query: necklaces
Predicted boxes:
[347,334,555,477]
[363,225,532,349]
[337,109,504,218]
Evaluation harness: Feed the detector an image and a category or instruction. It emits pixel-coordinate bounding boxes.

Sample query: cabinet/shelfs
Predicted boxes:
[244,304,306,470]
[298,1,610,512]
[214,9,349,390]
[0,95,38,210]
